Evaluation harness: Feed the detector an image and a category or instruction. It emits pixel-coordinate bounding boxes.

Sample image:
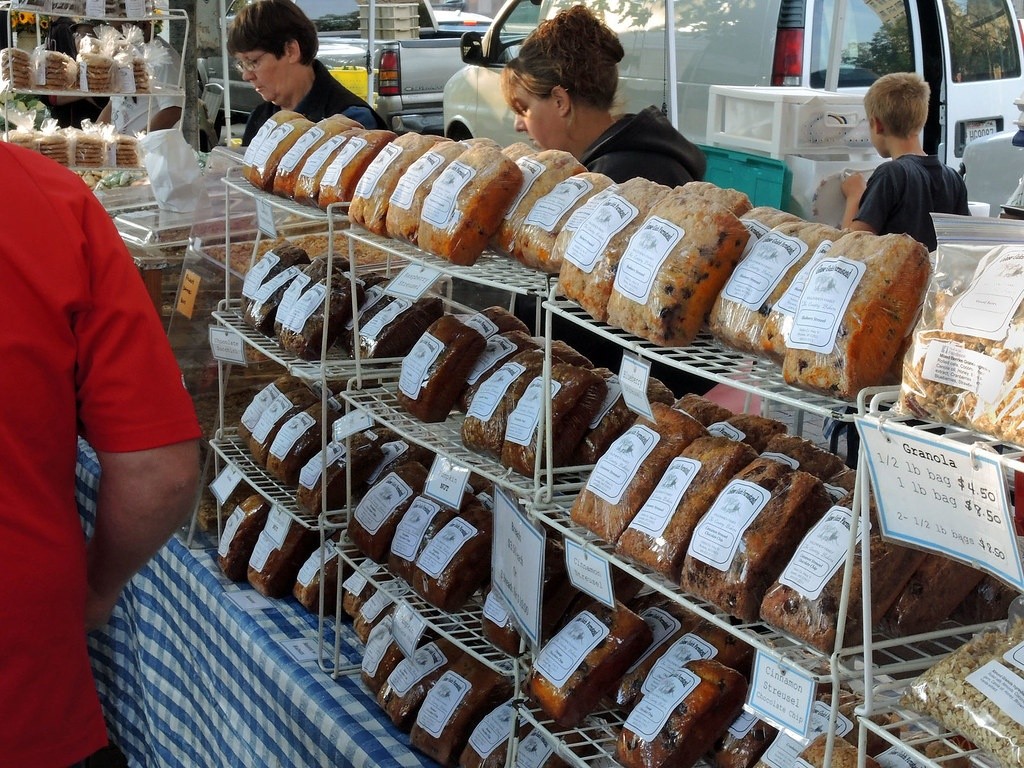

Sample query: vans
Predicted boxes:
[439,0,1023,211]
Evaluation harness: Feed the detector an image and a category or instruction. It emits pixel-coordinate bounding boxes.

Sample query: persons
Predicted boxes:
[227,0,390,147]
[837,72,971,254]
[506,4,708,396]
[0,139,202,768]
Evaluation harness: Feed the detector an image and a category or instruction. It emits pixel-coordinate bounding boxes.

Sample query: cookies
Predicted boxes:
[2,44,153,168]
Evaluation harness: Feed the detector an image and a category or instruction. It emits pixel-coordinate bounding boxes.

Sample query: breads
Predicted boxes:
[213,109,1017,768]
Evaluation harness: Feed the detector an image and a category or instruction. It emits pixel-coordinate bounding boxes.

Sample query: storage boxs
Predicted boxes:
[357,4,420,40]
[329,65,380,111]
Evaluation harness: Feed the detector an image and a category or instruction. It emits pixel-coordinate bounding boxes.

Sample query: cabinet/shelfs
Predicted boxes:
[0,0,190,171]
[705,83,889,227]
[188,166,1024,768]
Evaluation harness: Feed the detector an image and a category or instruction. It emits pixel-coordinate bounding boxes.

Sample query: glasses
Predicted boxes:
[236,51,270,72]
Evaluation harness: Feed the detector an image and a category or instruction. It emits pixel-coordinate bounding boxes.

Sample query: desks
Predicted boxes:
[73,390,465,768]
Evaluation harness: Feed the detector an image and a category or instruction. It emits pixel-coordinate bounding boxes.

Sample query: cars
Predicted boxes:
[433,10,497,28]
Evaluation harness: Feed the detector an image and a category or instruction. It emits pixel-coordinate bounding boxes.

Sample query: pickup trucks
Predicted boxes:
[187,0,532,156]
